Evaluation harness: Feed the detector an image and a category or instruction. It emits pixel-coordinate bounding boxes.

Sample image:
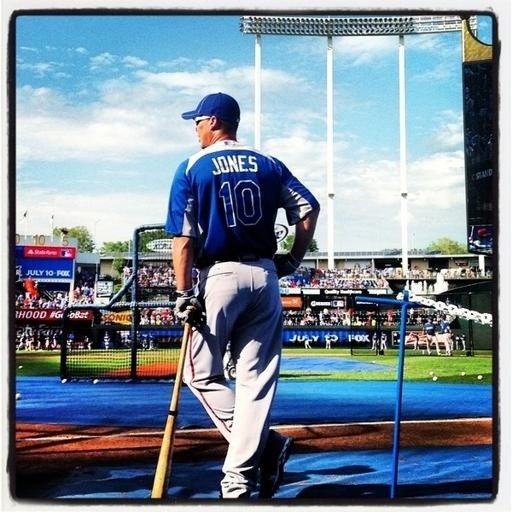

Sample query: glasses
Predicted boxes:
[190,115,212,127]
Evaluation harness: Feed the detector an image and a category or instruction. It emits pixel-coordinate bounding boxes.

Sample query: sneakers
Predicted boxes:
[257,429,295,497]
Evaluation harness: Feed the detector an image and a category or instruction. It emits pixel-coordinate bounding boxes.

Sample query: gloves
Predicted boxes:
[172,289,203,325]
[272,251,302,280]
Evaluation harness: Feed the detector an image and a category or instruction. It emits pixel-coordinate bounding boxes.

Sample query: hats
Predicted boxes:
[181,92,241,125]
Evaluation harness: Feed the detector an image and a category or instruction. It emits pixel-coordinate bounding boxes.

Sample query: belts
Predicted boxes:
[197,253,261,271]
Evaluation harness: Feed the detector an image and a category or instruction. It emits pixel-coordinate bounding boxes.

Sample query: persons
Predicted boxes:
[15,265,195,351]
[163,92,320,499]
[278,265,493,357]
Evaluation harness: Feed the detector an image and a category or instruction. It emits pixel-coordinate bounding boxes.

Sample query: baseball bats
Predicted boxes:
[151,305,197,500]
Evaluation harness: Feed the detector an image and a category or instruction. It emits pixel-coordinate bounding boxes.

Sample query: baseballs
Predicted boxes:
[93,378,99,384]
[15,393,22,400]
[18,366,23,369]
[478,374,483,379]
[433,377,437,381]
[461,372,466,376]
[429,371,433,375]
[61,378,67,383]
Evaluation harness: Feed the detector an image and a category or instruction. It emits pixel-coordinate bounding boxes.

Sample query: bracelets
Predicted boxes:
[288,253,301,271]
